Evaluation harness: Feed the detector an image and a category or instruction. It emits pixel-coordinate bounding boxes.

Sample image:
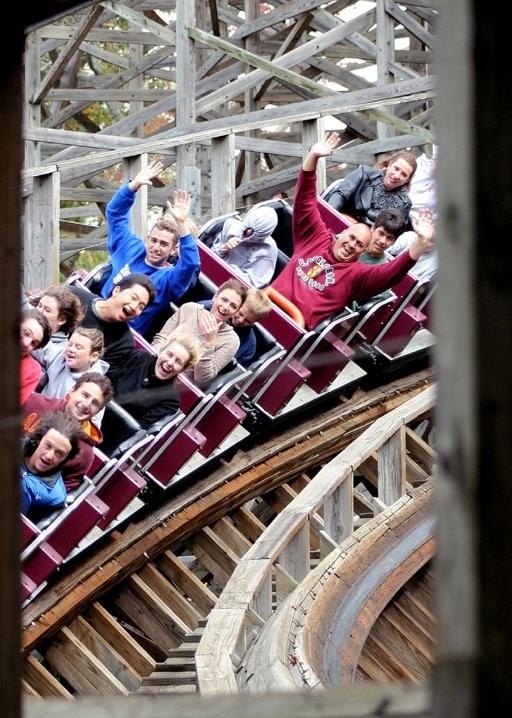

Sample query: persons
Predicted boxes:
[150,277,249,387]
[202,288,273,364]
[268,131,434,331]
[97,328,202,459]
[324,144,436,286]
[100,159,202,336]
[64,272,157,358]
[210,206,279,287]
[18,287,116,518]
